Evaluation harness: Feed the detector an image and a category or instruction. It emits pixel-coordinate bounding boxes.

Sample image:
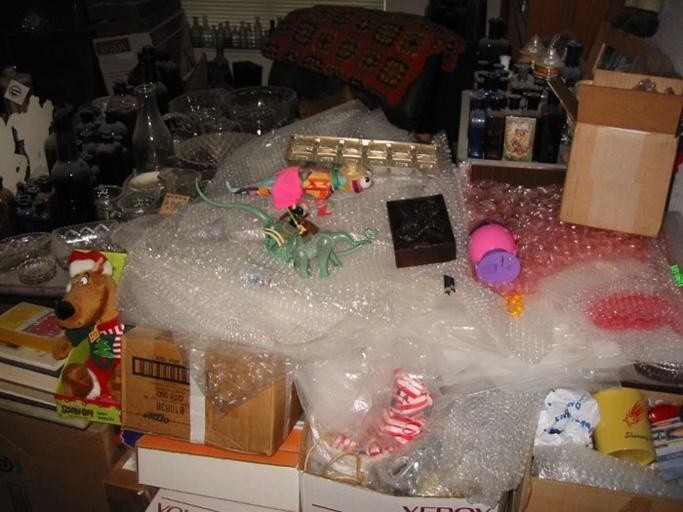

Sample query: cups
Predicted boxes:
[117,190,160,221]
[93,186,124,220]
[591,388,657,466]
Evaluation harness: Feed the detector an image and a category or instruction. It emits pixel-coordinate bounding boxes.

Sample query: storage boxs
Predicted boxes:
[120,325,304,457]
[300,467,514,512]
[546,69,682,240]
[102,447,159,512]
[0,410,127,512]
[511,387,683,512]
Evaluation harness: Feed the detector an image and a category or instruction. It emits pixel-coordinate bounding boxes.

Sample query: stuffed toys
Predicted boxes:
[50,249,129,400]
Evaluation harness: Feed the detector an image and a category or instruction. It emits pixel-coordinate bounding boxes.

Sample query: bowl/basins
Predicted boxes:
[222,85,298,128]
[168,89,227,120]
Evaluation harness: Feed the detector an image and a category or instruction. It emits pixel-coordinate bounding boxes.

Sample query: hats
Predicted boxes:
[68,249,112,278]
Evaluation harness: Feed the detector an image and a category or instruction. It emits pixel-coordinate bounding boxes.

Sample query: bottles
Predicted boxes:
[132,84,173,171]
[191,15,276,48]
[13,103,130,233]
[467,15,583,164]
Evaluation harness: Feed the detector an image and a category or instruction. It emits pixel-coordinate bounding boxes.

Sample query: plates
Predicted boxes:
[176,131,257,169]
[0,231,50,270]
